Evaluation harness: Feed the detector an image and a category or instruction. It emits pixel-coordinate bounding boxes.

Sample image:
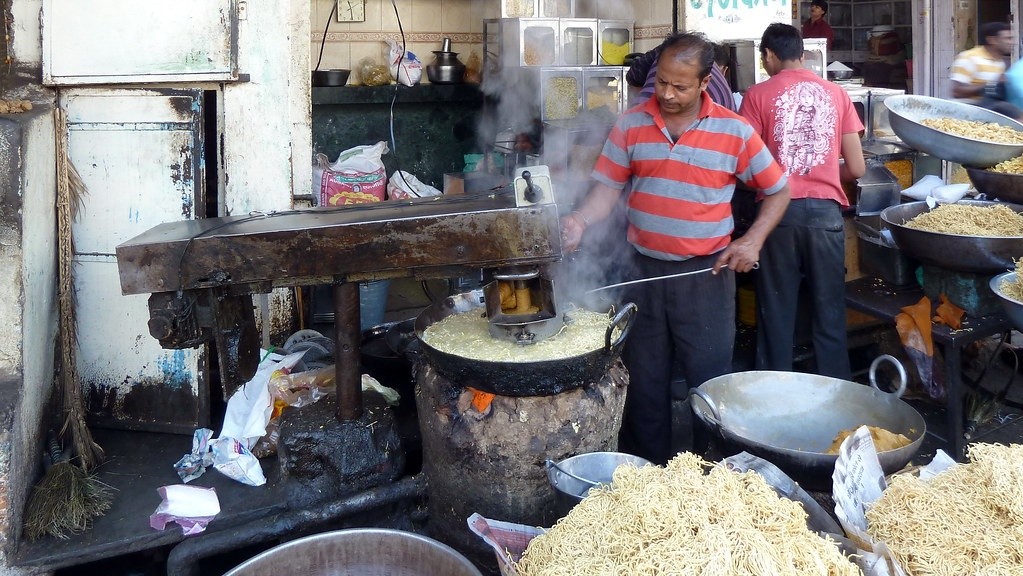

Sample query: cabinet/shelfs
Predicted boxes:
[797,0,912,63]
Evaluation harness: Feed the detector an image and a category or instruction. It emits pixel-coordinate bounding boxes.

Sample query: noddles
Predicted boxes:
[421,301,622,362]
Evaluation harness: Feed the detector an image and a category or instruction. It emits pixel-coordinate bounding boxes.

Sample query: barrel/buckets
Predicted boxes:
[223,528,481,576]
[359,279,389,331]
[544,451,654,516]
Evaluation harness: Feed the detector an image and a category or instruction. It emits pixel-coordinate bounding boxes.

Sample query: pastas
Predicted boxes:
[861,441,1023,576]
[504,448,866,575]
[999,256,1023,305]
[899,203,1023,238]
[543,77,578,119]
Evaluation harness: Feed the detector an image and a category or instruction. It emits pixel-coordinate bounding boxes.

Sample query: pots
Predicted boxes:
[414,280,638,395]
[687,355,927,493]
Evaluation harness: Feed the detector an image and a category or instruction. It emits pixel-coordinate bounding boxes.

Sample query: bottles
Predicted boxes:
[426,52,465,84]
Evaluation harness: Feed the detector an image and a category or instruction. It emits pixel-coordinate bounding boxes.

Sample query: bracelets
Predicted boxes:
[572,209,593,227]
[982,82,999,99]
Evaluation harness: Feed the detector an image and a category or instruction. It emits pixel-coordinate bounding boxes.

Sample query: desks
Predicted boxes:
[845,276,1023,464]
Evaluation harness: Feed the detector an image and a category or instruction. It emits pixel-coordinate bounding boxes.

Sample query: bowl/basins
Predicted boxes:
[961,165,1023,204]
[883,94,1023,165]
[311,68,352,87]
[827,70,854,80]
[869,30,889,37]
[988,270,1023,334]
[879,199,1023,270]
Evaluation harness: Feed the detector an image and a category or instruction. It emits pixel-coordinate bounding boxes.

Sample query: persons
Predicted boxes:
[803,0,833,48]
[738,23,867,384]
[949,20,1023,122]
[559,28,792,461]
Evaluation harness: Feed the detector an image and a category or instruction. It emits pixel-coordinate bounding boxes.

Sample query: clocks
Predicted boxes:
[336,0,365,22]
